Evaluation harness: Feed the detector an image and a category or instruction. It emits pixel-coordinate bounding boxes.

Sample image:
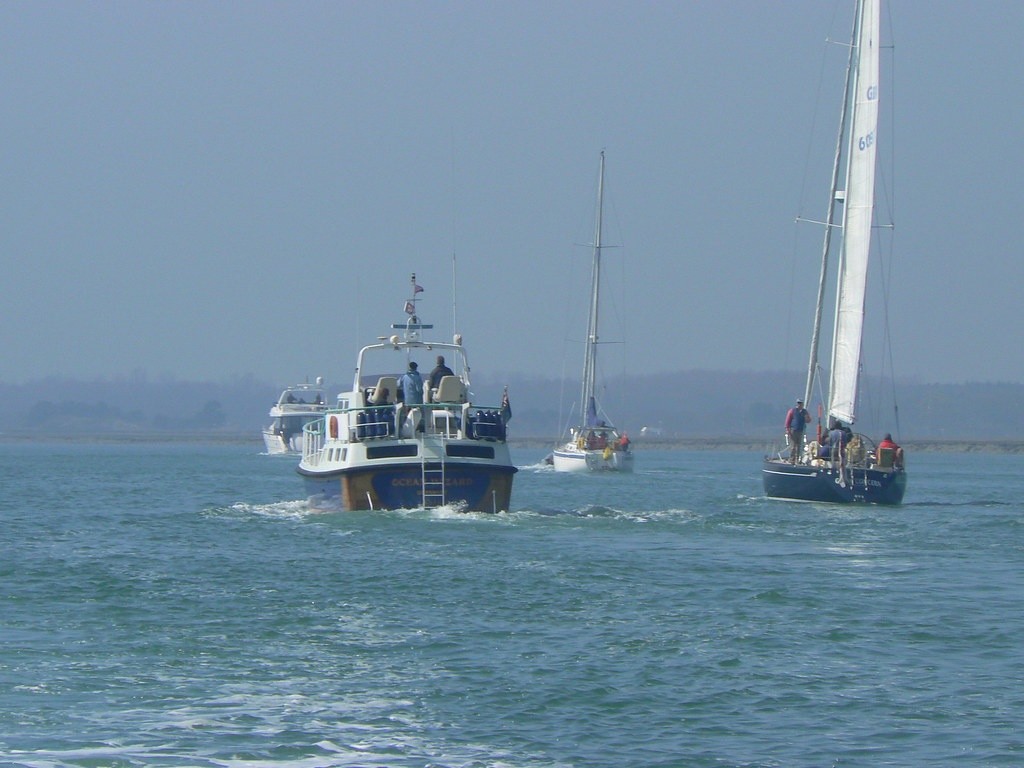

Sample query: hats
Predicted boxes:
[796,398,804,402]
[436,356,445,364]
[409,362,418,368]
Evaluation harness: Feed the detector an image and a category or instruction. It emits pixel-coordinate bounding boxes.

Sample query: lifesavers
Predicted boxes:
[330,416,337,438]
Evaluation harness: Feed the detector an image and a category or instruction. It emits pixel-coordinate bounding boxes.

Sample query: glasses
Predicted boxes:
[797,402,802,404]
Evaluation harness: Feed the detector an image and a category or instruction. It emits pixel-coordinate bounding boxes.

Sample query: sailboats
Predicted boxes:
[763,0,908,506]
[554,146,635,475]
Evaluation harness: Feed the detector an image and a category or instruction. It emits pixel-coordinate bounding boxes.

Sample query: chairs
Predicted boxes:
[365,376,397,403]
[431,375,461,401]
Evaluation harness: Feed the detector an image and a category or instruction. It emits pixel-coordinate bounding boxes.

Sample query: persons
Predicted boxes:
[876,433,900,467]
[298,398,306,403]
[784,399,811,464]
[818,421,853,457]
[621,433,630,450]
[587,431,608,449]
[429,356,454,388]
[316,393,321,404]
[397,362,423,403]
[288,394,296,403]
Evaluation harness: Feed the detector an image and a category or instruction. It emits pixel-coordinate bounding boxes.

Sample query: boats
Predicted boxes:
[258,374,336,457]
[296,252,519,514]
